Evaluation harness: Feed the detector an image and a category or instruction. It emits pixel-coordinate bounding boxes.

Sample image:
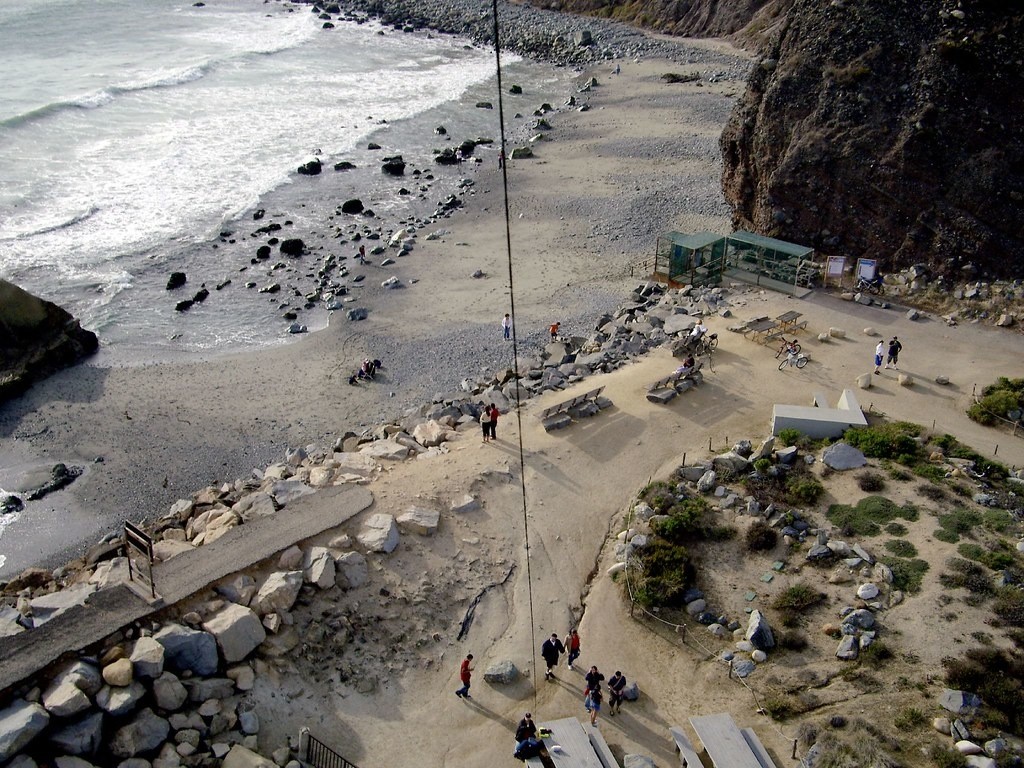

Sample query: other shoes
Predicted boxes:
[490,437,496,440]
[482,442,486,443]
[485,441,489,443]
[885,364,889,368]
[610,711,614,716]
[893,366,898,370]
[549,672,555,678]
[591,721,597,727]
[507,338,511,340]
[503,338,505,341]
[545,672,549,680]
[463,695,471,697]
[874,371,880,375]
[455,691,462,698]
[568,663,573,670]
[587,710,591,713]
[616,708,621,714]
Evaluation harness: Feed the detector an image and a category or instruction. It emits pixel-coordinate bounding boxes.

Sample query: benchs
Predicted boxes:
[531,385,607,419]
[669,727,704,768]
[740,727,777,768]
[642,363,704,392]
[581,721,621,768]
[516,742,544,768]
[790,321,809,330]
[764,331,784,340]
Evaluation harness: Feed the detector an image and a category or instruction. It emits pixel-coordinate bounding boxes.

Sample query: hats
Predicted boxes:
[526,712,531,718]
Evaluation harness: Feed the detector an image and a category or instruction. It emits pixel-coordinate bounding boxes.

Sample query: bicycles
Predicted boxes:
[779,349,808,371]
[695,334,718,356]
[775,337,801,358]
[698,351,715,373]
[853,276,884,296]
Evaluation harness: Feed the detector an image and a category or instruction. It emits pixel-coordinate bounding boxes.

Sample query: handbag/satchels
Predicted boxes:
[608,686,612,701]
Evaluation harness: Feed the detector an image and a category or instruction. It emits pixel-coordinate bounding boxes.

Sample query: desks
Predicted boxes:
[535,716,604,768]
[776,311,803,335]
[748,321,780,343]
[687,713,762,768]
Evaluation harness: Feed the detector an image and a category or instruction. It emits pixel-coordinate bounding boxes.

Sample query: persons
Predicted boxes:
[541,633,565,679]
[683,353,695,368]
[885,335,902,370]
[489,403,501,440]
[564,628,581,671]
[584,666,605,713]
[479,405,491,443]
[607,671,626,718]
[501,313,513,341]
[874,339,885,375]
[589,684,605,727]
[515,712,537,743]
[548,321,561,341]
[454,654,475,699]
[691,320,707,337]
